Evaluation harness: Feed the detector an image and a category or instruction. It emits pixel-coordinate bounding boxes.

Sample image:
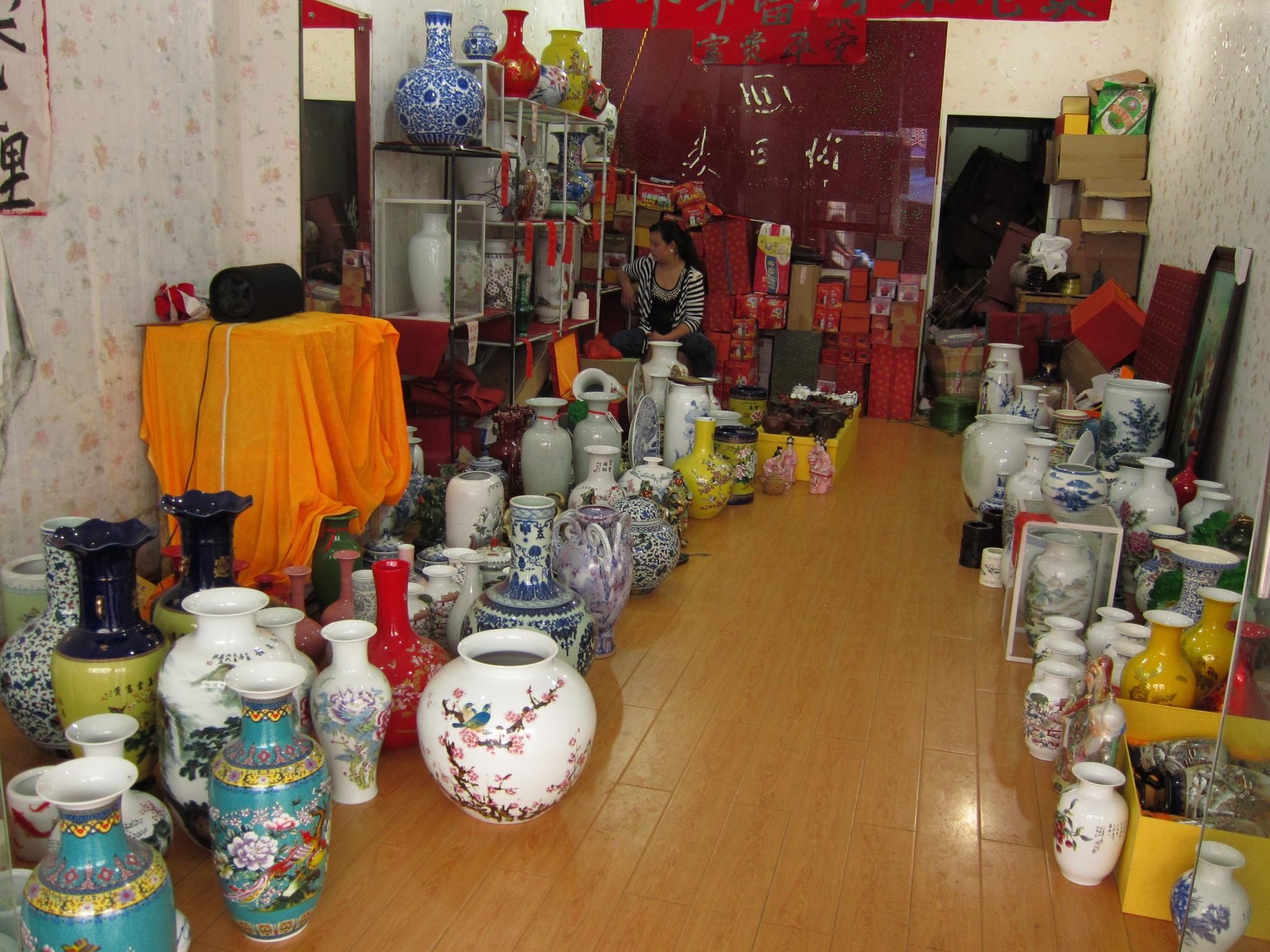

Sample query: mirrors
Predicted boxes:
[299,0,372,317]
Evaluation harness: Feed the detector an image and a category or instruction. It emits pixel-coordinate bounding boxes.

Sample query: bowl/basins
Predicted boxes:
[545,201,577,217]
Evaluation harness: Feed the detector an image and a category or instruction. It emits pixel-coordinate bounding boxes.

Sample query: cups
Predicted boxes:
[1,867,34,952]
[6,764,60,863]
[960,520,994,568]
[981,508,1006,547]
[3,554,47,642]
[572,298,591,318]
[978,547,1007,588]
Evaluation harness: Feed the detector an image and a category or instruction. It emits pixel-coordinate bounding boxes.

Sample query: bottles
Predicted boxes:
[0,339,769,952]
[393,10,618,321]
[961,338,1270,951]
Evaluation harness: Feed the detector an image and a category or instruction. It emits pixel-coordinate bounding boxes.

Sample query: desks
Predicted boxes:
[139,311,413,606]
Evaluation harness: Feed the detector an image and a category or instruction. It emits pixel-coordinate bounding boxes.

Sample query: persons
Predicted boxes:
[609,220,716,377]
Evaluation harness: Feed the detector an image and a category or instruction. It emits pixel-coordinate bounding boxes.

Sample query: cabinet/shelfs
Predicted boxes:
[373,59,637,466]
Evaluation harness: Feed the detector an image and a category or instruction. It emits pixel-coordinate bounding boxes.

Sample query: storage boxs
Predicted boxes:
[1115,697,1270,941]
[581,70,1251,486]
[304,193,373,307]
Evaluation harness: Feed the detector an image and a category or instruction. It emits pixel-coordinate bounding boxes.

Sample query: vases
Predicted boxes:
[393,9,618,323]
[0,341,1270,952]
